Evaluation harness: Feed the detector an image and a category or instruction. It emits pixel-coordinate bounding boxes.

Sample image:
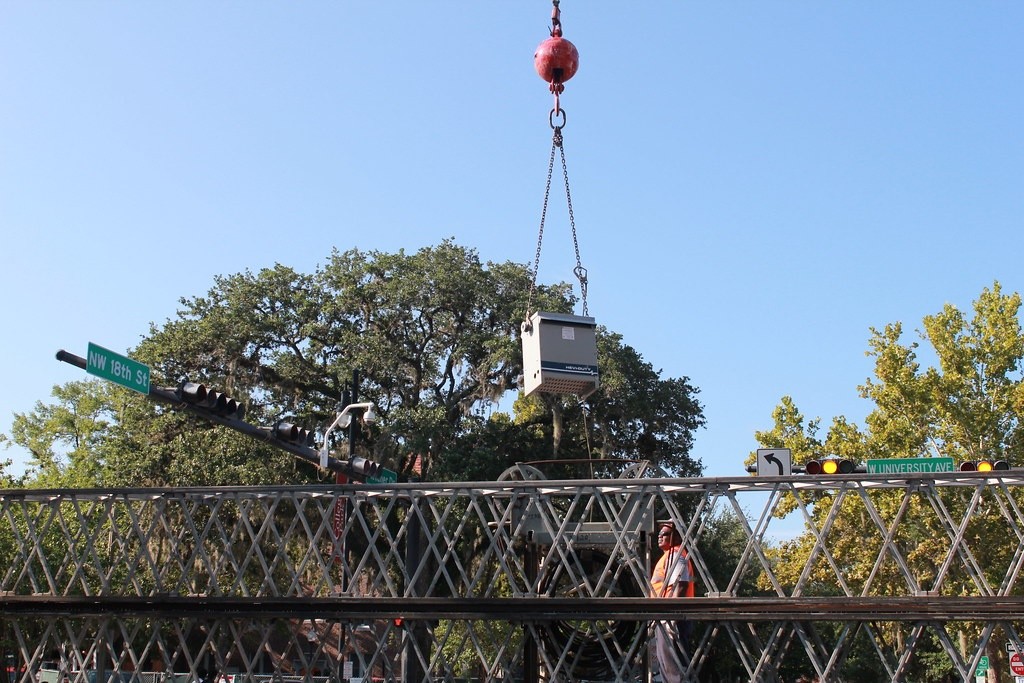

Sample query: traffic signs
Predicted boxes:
[364,466,398,488]
[756,449,791,476]
[84,341,150,396]
[866,458,954,476]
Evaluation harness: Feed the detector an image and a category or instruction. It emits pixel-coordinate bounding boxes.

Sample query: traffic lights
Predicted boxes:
[274,424,315,447]
[177,382,246,422]
[351,457,383,478]
[804,459,856,475]
[959,462,1011,472]
[394,618,402,627]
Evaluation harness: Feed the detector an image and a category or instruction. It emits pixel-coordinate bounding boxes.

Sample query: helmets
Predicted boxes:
[659,518,676,530]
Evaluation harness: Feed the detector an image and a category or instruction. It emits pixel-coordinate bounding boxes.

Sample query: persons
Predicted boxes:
[650,518,701,683]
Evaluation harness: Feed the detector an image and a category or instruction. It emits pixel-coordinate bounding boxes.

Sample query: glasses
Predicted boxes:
[659,531,671,536]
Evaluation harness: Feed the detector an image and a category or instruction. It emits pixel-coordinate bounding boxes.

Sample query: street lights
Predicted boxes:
[323,371,361,683]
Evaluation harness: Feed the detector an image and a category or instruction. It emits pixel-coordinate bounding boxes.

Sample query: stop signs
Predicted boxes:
[1008,651,1024,676]
